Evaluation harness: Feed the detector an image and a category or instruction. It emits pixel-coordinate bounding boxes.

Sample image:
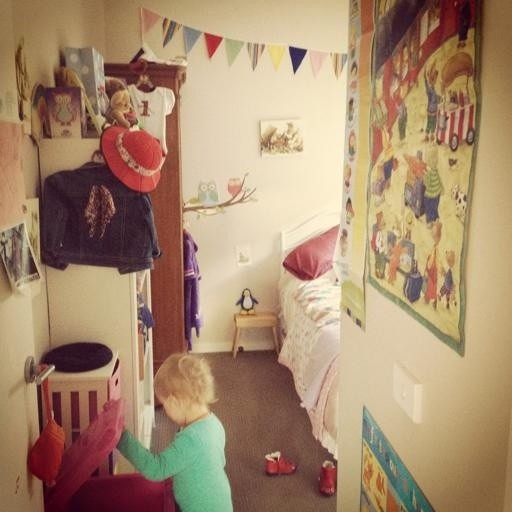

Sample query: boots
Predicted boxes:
[265,453,297,475]
[319,460,336,495]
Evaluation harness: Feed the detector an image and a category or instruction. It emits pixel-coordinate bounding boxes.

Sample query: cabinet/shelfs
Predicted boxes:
[103,61,188,410]
[37,138,158,473]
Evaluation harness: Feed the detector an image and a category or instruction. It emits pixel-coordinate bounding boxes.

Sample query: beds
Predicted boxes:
[277,211,340,460]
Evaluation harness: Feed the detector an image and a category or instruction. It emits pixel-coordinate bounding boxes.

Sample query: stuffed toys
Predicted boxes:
[106,79,138,129]
[235,289,259,315]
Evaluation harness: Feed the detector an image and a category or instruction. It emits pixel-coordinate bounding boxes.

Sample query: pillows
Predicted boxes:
[283,224,340,281]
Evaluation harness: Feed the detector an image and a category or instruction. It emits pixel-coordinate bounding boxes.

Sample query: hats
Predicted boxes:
[102,125,164,194]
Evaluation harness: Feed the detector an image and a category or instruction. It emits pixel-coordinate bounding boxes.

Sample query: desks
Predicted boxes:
[232,312,279,360]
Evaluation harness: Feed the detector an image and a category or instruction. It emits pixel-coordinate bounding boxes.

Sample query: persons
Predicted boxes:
[117,352,234,512]
[371,1,471,310]
